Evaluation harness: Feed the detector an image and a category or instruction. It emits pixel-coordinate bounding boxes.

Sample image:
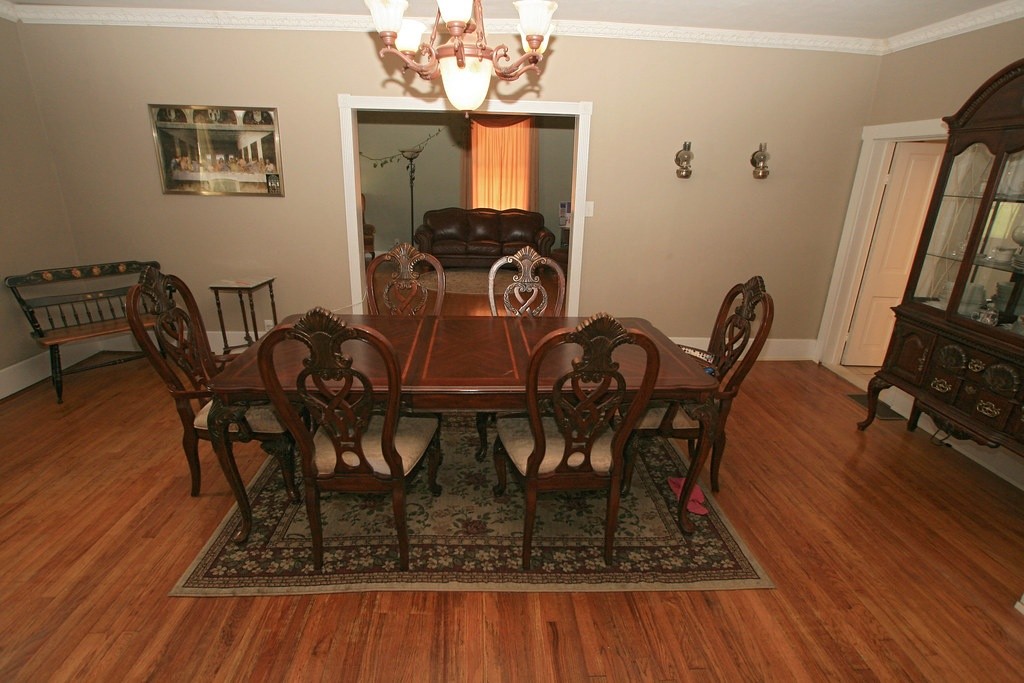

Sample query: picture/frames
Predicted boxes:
[147,104,285,198]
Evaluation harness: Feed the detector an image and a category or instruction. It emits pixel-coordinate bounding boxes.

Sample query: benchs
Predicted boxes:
[5,260,178,405]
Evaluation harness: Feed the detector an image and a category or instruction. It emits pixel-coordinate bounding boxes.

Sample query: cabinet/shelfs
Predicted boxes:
[856,57,1024,459]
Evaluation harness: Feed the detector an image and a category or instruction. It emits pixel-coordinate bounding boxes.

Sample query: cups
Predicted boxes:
[994,246,1018,263]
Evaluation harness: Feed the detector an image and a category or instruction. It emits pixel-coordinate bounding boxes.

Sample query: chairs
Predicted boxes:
[126,265,311,504]
[493,312,659,572]
[475,246,565,462]
[257,307,443,572]
[551,226,570,283]
[362,193,376,261]
[365,242,445,466]
[605,275,775,499]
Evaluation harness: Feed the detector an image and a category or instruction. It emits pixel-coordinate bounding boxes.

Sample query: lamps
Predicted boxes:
[750,142,770,180]
[399,148,422,247]
[674,142,694,179]
[364,0,558,118]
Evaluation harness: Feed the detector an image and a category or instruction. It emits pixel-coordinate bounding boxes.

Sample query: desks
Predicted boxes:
[210,276,277,370]
[207,313,721,545]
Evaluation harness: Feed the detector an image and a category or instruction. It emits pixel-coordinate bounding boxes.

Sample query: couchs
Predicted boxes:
[414,207,555,274]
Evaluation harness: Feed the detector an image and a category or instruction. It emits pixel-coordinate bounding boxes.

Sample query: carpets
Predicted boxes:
[416,268,523,296]
[168,451,776,597]
